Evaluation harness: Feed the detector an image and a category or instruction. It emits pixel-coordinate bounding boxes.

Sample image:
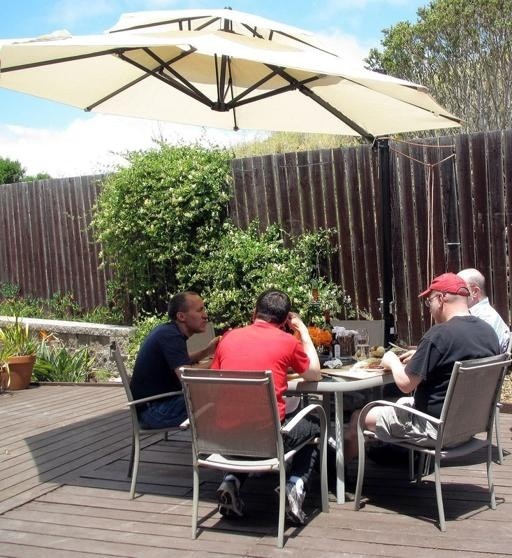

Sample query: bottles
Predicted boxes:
[329,333,339,360]
[323,312,334,331]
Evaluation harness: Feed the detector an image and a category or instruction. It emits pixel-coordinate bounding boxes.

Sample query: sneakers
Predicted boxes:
[273,479,308,526]
[215,477,248,518]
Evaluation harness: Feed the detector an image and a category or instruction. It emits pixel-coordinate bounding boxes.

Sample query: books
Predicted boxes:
[322,358,393,379]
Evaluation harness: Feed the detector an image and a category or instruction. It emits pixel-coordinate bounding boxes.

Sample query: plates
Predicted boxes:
[356,366,385,372]
[372,351,406,357]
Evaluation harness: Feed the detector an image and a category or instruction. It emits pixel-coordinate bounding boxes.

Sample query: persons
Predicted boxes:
[452,267,511,352]
[129,291,224,426]
[313,272,500,496]
[209,289,321,525]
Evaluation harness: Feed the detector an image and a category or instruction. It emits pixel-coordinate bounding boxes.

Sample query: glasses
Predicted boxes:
[424,293,440,307]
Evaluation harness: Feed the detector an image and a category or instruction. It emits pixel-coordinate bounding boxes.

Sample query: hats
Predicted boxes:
[416,272,471,298]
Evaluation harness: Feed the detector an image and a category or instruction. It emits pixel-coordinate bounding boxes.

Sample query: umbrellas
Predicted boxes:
[0,8,464,347]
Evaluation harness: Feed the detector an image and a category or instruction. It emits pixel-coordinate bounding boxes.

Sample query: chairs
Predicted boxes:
[353,353,512,531]
[110,340,185,501]
[176,366,330,547]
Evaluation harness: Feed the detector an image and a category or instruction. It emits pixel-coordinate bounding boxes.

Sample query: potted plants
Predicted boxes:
[0,311,37,391]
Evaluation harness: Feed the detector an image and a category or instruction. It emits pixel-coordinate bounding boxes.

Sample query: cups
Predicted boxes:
[354,328,369,359]
